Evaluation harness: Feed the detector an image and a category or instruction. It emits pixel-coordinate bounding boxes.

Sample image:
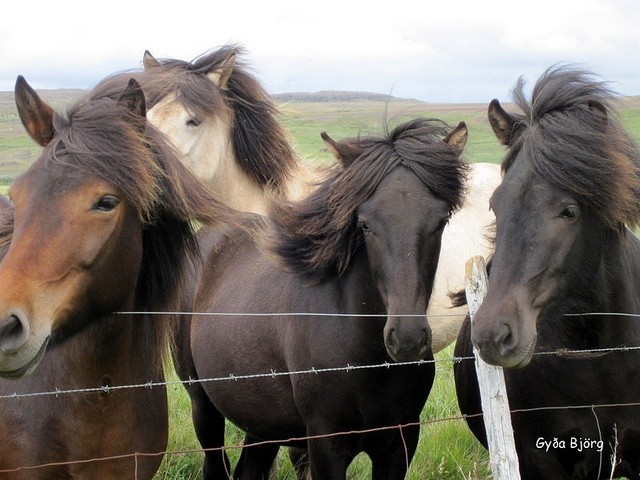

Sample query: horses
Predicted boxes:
[170,86,470,475]
[452,59,638,476]
[140,47,510,353]
[0,76,235,474]
